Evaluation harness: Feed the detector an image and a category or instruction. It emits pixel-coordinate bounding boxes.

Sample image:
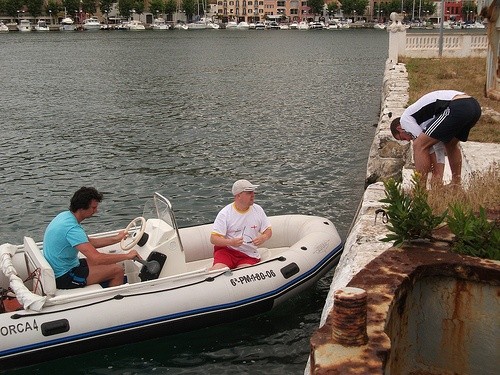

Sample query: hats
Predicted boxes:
[231,180,260,196]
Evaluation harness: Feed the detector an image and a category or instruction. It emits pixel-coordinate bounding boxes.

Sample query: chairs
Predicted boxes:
[22,236,103,297]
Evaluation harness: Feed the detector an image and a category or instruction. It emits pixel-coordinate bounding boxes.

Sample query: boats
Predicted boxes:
[0,19,10,33]
[150,18,169,31]
[6,22,19,31]
[81,18,102,30]
[404,21,486,30]
[18,20,33,33]
[0,191,343,366]
[127,19,146,30]
[35,20,50,31]
[173,20,353,30]
[59,17,76,31]
[374,23,386,30]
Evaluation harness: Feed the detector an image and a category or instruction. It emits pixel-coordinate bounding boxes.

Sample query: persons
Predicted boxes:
[210,179,272,270]
[44,187,141,289]
[390,90,481,194]
